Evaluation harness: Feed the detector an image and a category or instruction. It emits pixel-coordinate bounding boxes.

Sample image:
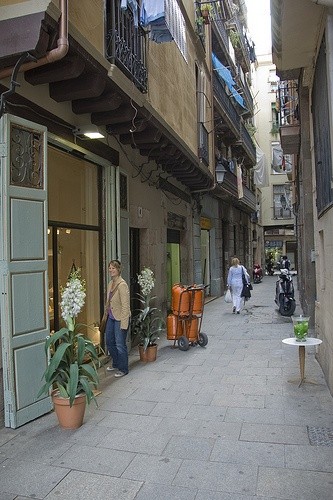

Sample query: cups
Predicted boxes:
[291,315,310,342]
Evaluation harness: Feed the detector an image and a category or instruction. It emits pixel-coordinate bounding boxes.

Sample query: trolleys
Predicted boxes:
[171,284,211,351]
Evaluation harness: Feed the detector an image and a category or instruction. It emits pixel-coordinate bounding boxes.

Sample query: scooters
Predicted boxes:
[265,265,274,276]
[274,268,298,316]
[253,264,263,284]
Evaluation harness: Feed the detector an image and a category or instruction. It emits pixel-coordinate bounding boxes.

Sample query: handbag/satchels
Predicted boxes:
[224,289,232,303]
[98,312,109,333]
[242,274,246,284]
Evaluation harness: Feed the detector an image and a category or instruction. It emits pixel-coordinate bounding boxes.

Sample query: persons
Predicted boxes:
[227,258,250,314]
[100,260,130,377]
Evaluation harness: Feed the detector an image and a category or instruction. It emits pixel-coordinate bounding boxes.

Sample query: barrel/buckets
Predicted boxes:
[167,284,204,342]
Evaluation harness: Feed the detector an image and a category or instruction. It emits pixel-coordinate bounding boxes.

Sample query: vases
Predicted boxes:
[50,389,87,429]
[139,343,158,362]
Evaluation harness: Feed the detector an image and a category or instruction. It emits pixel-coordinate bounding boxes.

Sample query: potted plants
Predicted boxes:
[200,4,215,25]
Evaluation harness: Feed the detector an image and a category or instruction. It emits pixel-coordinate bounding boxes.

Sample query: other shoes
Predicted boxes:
[236,310,240,314]
[115,371,128,377]
[107,366,116,371]
[233,307,235,312]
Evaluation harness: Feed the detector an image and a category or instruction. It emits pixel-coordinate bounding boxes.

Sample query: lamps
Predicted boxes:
[71,123,106,142]
[280,193,293,213]
[215,161,227,188]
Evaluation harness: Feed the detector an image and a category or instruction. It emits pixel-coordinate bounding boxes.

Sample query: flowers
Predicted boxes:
[131,267,167,354]
[36,267,108,407]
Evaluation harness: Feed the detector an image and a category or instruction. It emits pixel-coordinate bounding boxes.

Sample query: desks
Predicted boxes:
[282,338,323,389]
